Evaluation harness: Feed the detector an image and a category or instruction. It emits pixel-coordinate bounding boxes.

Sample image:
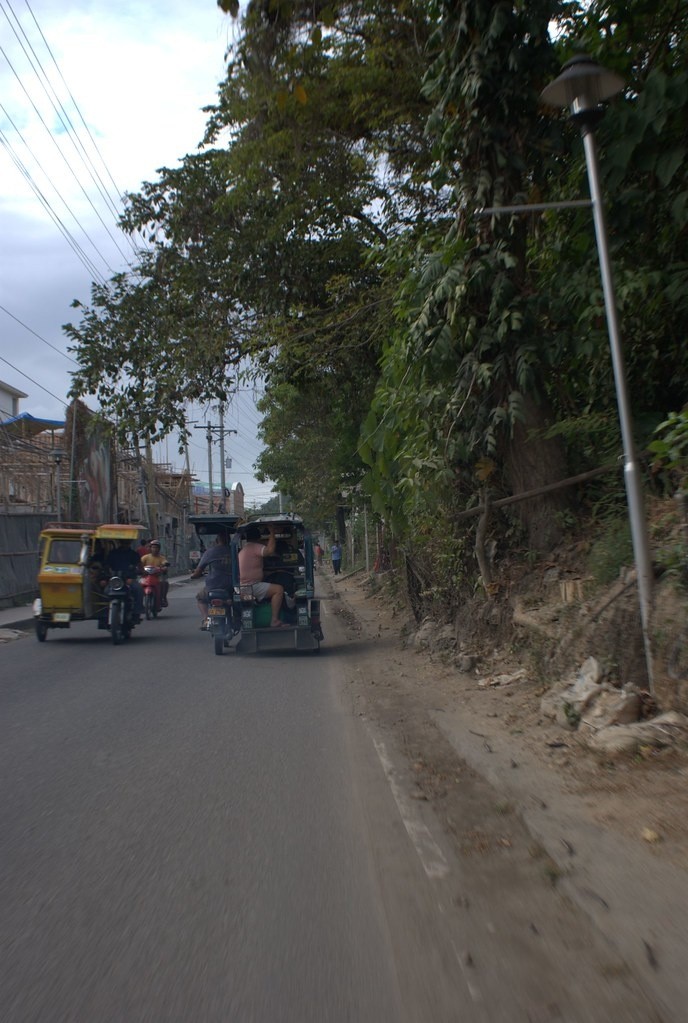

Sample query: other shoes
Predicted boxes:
[126,612,141,625]
[98,614,110,628]
[200,619,210,631]
[161,598,168,607]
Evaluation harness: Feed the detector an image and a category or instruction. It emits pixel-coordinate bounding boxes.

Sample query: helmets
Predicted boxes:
[150,540,161,547]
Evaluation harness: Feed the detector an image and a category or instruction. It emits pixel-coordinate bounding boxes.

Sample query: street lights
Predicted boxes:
[49,447,70,530]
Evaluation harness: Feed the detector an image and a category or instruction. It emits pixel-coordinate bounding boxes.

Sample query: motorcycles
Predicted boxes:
[136,562,171,621]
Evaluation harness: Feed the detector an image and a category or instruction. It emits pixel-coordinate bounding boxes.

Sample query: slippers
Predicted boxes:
[270,621,290,628]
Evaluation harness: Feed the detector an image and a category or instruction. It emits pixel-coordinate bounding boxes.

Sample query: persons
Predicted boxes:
[56,545,69,563]
[89,539,151,630]
[332,540,343,575]
[317,544,323,566]
[238,523,290,628]
[138,540,169,607]
[191,530,242,631]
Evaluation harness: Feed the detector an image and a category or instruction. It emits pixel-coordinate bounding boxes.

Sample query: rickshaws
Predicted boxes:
[31,523,150,648]
[186,512,325,657]
